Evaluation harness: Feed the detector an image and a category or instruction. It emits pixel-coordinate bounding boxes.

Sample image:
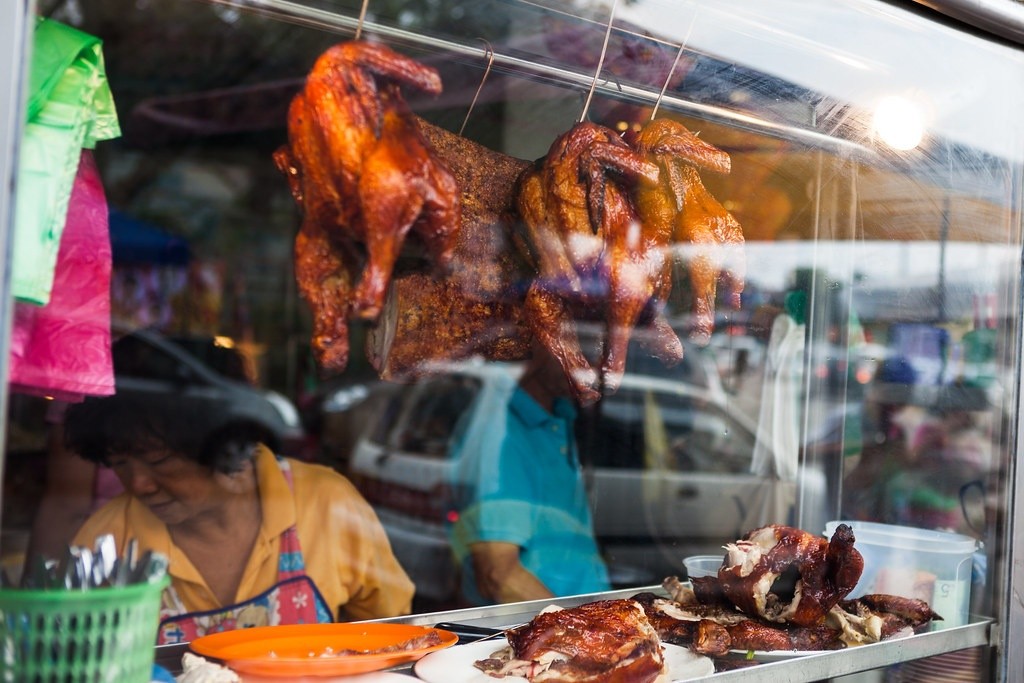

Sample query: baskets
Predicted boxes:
[0,575,172,683]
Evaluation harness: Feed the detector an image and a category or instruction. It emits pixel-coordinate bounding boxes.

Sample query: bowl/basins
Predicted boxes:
[683,555,726,591]
[822,520,979,635]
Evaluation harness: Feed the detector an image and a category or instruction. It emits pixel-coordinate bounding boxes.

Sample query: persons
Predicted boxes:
[439,346,614,608]
[22,318,419,641]
[803,361,1005,615]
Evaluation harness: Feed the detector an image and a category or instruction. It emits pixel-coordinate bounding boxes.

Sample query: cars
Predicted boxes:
[319,306,948,492]
[11,301,312,499]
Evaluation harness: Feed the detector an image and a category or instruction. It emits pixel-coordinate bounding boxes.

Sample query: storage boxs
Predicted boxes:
[683,555,725,589]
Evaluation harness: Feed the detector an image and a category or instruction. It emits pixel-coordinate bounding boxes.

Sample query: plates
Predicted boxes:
[888,645,982,683]
[187,623,459,676]
[416,639,714,683]
[250,670,426,683]
[725,624,914,662]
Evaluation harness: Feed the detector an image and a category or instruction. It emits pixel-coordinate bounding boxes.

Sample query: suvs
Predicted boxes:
[346,358,814,610]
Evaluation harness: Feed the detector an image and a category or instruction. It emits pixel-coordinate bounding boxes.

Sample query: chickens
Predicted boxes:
[518,119,747,407]
[273,41,461,369]
